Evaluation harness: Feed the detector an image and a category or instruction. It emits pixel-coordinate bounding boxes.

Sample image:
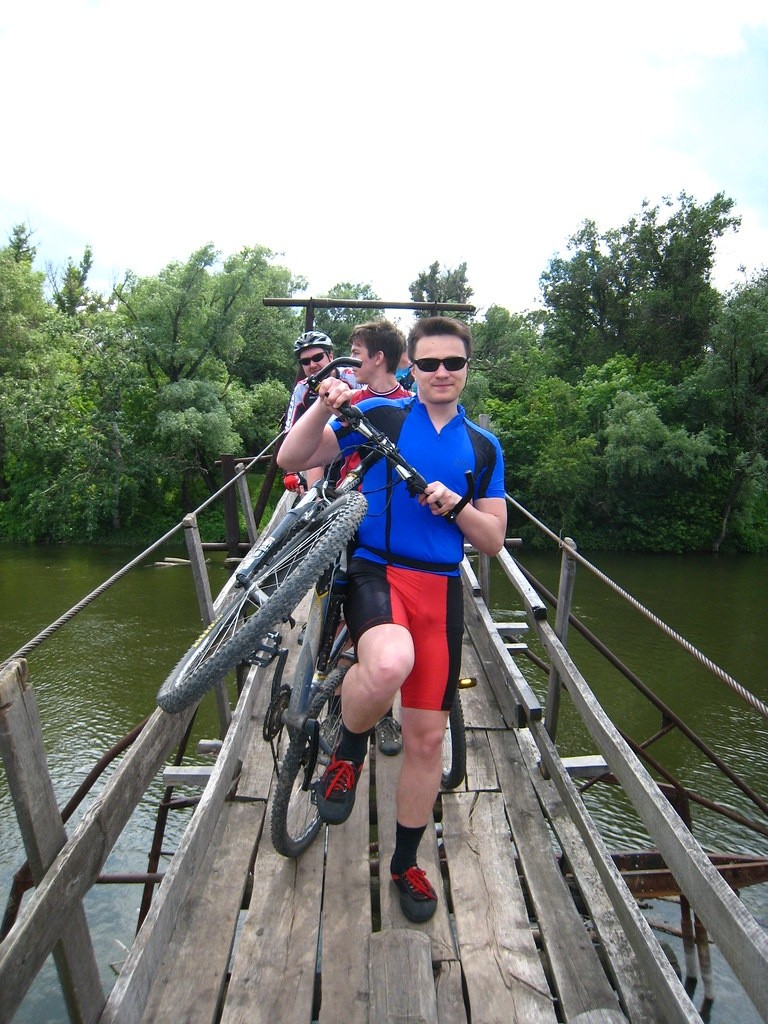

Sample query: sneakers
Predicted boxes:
[390,853,438,923]
[317,733,367,825]
[376,716,405,755]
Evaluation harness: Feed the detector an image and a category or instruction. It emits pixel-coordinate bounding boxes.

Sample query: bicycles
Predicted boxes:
[157,356,476,857]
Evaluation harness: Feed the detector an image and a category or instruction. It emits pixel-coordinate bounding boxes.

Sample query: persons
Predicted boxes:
[283,319,418,757]
[277,316,506,922]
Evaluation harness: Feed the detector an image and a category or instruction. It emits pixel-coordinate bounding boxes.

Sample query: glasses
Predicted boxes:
[408,356,472,371]
[298,351,327,365]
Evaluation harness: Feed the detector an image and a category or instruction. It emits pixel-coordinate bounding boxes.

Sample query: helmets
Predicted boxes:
[294,331,334,355]
